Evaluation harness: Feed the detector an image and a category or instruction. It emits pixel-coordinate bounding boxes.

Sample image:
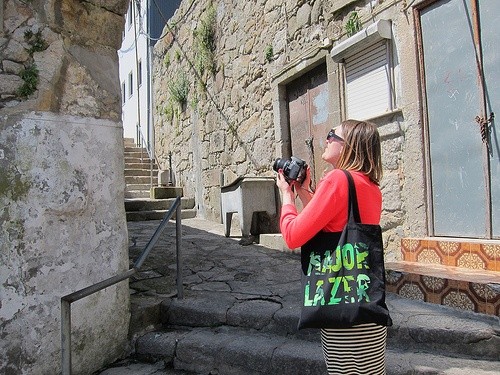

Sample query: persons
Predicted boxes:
[276,119,388,375]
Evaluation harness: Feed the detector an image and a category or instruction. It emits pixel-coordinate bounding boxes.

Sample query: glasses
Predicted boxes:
[327,129,344,141]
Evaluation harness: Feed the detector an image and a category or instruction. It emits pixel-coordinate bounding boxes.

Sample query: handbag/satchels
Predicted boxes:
[296,169,393,330]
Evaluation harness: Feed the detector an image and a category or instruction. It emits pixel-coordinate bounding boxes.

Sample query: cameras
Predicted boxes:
[272,156,308,183]
